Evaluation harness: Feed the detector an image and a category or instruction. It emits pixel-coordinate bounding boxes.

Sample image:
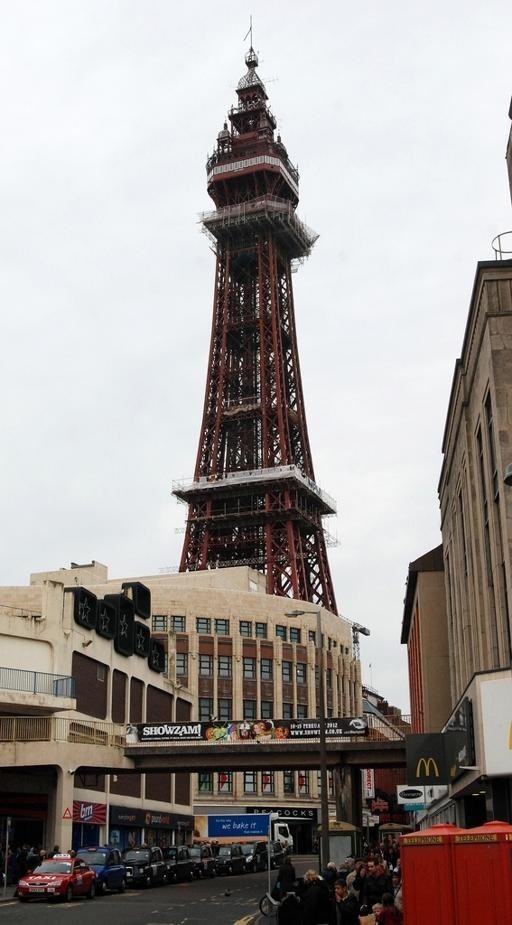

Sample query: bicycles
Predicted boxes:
[259,895,279,917]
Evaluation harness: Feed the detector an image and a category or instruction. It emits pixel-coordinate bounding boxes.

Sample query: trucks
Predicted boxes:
[194,812,294,855]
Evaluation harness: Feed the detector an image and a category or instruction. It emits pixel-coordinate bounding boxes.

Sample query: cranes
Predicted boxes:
[338,612,369,661]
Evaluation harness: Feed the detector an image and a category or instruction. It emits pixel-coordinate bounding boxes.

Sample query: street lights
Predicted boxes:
[283,610,330,864]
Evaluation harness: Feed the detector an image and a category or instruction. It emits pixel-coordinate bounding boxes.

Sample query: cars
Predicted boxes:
[17,841,287,903]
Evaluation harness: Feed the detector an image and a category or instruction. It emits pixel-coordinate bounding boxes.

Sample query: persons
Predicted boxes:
[225,720,290,740]
[0,843,60,879]
[268,834,403,924]
